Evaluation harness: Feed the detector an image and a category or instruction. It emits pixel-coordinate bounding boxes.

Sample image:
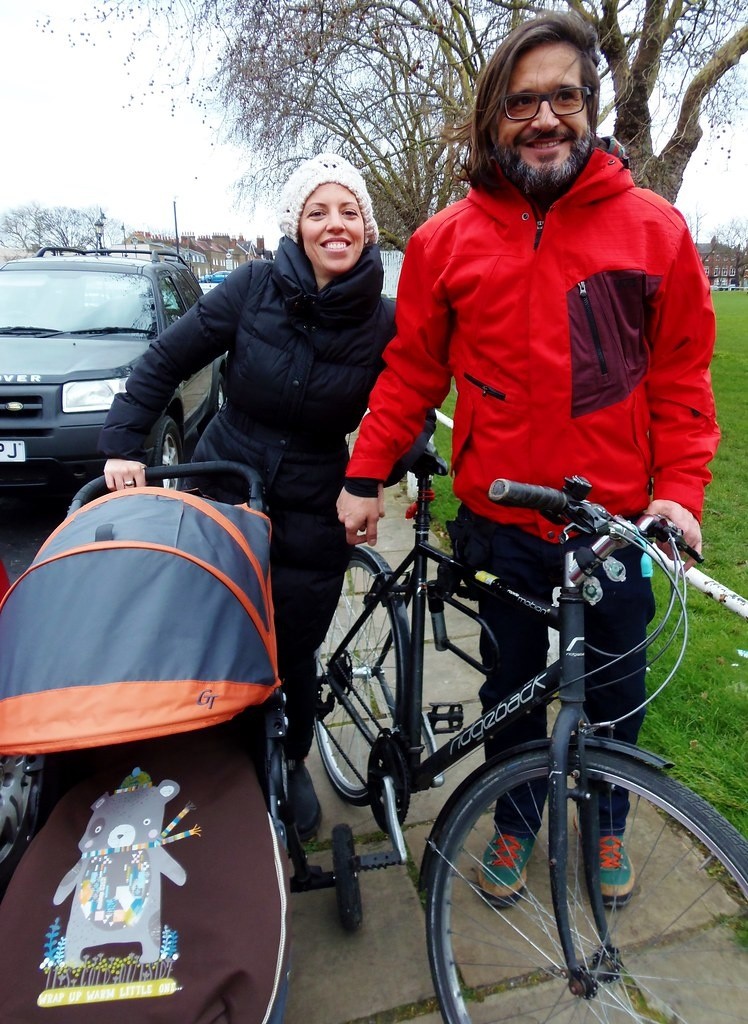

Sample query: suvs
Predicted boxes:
[0,244,224,492]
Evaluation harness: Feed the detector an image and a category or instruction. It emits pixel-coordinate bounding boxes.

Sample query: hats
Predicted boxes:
[279,152,379,251]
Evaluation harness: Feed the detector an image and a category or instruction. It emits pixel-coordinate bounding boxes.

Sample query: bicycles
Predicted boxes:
[305,437,748,1024]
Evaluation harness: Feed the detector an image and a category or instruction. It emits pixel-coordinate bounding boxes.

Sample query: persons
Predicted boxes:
[95,153,428,843]
[337,13,722,908]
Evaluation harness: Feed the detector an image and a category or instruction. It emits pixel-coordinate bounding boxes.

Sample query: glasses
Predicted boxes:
[504,86,592,120]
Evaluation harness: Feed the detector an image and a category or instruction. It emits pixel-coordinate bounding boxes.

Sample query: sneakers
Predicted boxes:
[477,827,535,907]
[574,810,634,909]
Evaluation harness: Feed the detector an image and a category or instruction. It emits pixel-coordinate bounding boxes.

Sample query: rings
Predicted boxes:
[124,481,134,486]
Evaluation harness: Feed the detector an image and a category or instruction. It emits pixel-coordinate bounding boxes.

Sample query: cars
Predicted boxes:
[197,269,232,295]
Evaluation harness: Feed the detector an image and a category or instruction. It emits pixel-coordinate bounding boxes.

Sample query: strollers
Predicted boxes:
[0,462,365,1024]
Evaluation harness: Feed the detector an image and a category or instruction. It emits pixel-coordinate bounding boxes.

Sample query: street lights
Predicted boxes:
[120,221,128,257]
[94,219,103,255]
[132,235,139,258]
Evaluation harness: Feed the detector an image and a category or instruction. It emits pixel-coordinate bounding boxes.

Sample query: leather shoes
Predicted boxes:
[290,764,320,840]
